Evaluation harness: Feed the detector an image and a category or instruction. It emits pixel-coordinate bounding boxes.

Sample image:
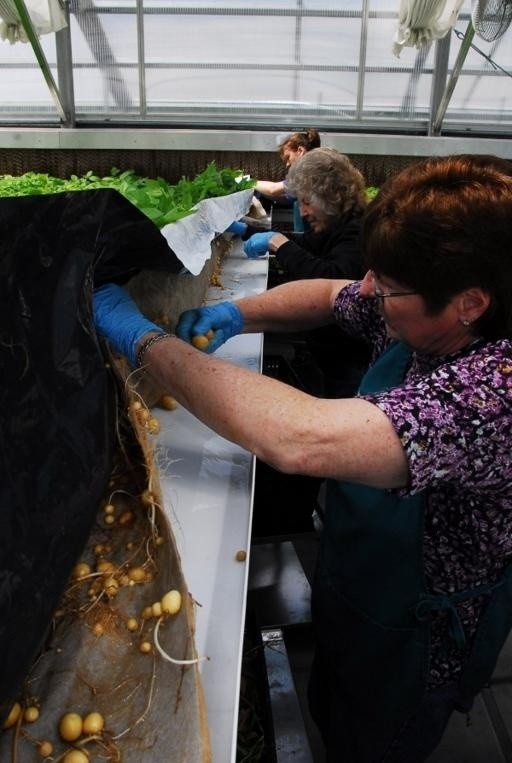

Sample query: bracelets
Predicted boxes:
[138,334,175,374]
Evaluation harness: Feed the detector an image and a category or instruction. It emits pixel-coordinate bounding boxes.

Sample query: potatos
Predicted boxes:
[4,703,103,763]
[131,330,213,434]
[74,506,183,652]
[237,551,246,560]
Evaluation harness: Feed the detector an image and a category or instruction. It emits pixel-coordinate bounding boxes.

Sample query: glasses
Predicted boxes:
[371,272,418,297]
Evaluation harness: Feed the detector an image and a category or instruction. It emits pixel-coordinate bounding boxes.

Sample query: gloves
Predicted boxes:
[91,283,163,368]
[227,220,248,236]
[177,301,244,354]
[243,232,278,258]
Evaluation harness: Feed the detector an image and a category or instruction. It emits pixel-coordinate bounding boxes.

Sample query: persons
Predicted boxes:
[233,128,322,234]
[231,149,371,537]
[93,155,512,763]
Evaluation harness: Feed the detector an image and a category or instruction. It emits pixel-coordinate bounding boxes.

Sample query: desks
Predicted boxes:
[0,195,273,763]
